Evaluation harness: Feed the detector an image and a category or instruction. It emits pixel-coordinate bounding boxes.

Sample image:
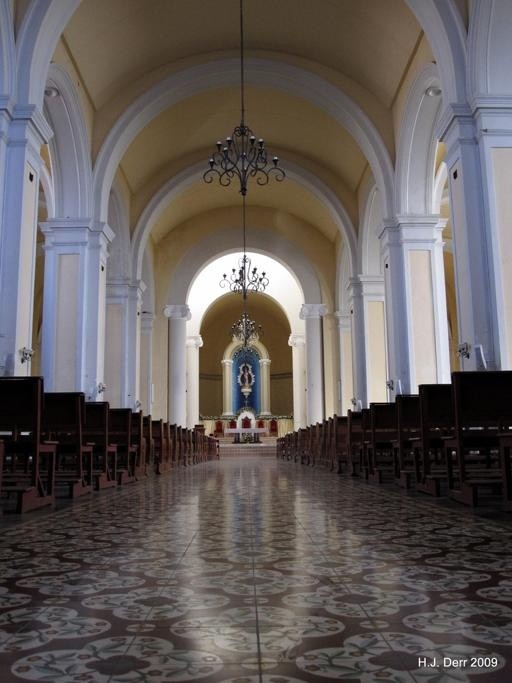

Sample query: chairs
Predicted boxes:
[0,377,225,513]
[276,370,512,522]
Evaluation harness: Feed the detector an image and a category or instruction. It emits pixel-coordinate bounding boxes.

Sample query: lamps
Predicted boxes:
[232,298,259,348]
[203,2,288,194]
[217,198,272,295]
